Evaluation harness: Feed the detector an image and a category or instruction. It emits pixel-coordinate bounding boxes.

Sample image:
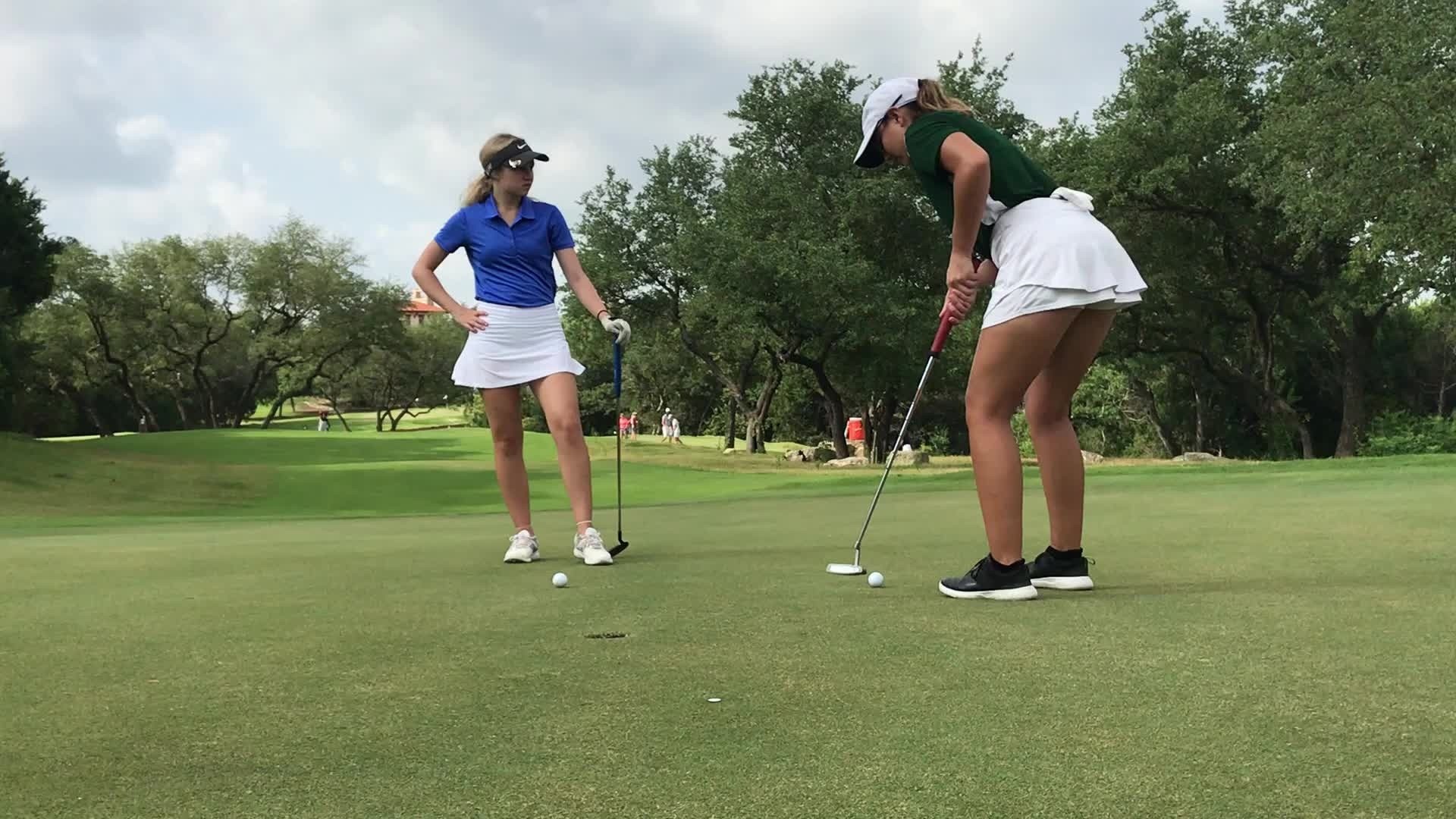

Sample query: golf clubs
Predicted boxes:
[824,307,955,575]
[606,334,629,558]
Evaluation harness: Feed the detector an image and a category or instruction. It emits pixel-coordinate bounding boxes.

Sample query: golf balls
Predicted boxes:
[552,572,568,587]
[867,572,884,587]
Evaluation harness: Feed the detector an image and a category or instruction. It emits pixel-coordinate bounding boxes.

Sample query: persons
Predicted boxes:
[662,408,683,445]
[412,133,631,566]
[854,72,1148,603]
[617,412,638,440]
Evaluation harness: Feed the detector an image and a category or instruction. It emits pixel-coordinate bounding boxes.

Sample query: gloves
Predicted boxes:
[603,315,631,345]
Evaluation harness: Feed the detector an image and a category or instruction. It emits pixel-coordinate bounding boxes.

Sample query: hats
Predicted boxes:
[855,78,922,168]
[665,408,670,412]
[483,139,547,176]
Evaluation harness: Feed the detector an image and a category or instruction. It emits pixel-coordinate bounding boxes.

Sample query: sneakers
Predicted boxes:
[938,555,1039,601]
[573,528,612,565]
[1021,552,1094,590]
[502,530,541,563]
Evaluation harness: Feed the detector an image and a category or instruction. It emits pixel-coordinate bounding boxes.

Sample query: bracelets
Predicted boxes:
[597,309,612,319]
